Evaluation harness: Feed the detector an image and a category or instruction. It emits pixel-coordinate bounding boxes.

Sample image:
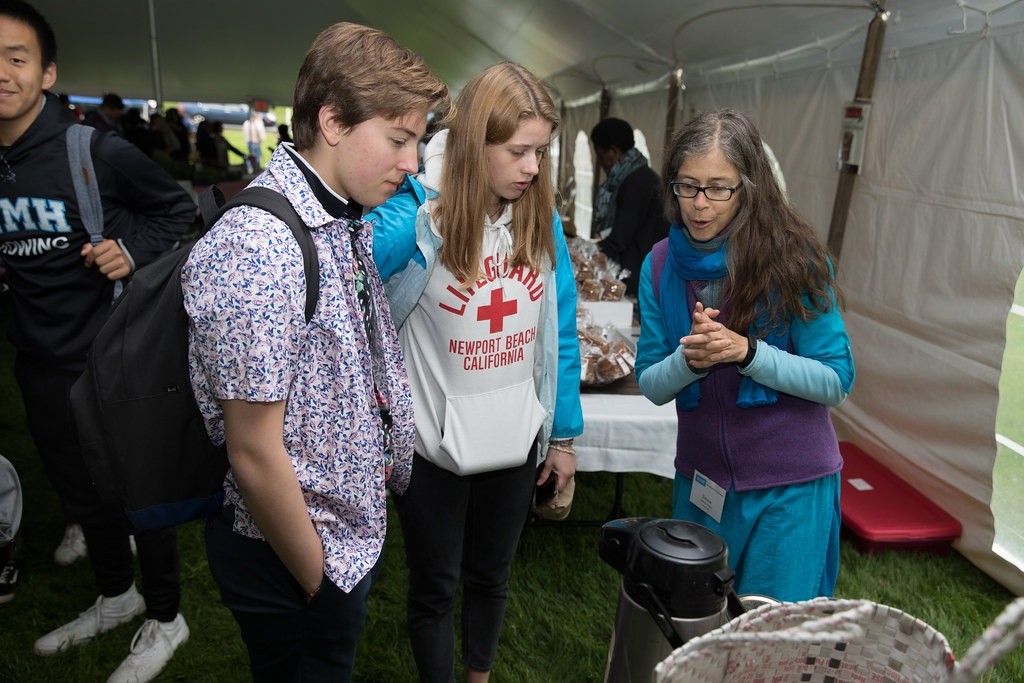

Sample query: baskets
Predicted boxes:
[655,597,1023,683]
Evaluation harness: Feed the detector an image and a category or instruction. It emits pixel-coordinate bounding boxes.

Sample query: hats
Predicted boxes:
[103,94,124,110]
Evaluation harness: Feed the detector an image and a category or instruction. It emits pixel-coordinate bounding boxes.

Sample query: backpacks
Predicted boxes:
[68,184,320,536]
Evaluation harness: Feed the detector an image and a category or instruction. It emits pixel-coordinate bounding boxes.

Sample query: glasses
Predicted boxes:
[671,179,743,201]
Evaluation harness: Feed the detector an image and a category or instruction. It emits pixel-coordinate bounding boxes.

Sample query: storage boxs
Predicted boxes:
[840,441,963,558]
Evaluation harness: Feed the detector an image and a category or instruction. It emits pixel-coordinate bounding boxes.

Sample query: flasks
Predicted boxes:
[598,516,737,683]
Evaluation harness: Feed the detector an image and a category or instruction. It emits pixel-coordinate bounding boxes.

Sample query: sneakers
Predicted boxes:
[33,582,148,656]
[106,613,188,683]
[0,557,24,603]
[54,524,87,566]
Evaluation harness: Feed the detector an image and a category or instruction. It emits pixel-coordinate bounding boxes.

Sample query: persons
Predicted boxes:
[182,20,460,683]
[242,113,267,168]
[636,106,858,604]
[588,117,669,295]
[362,60,586,683]
[84,92,247,183]
[268,123,294,153]
[0,0,197,683]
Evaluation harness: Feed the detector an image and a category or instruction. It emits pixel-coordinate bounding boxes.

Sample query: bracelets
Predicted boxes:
[548,444,577,455]
[740,334,756,368]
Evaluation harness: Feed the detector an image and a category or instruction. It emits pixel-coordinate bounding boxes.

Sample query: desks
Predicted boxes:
[573,394,678,517]
